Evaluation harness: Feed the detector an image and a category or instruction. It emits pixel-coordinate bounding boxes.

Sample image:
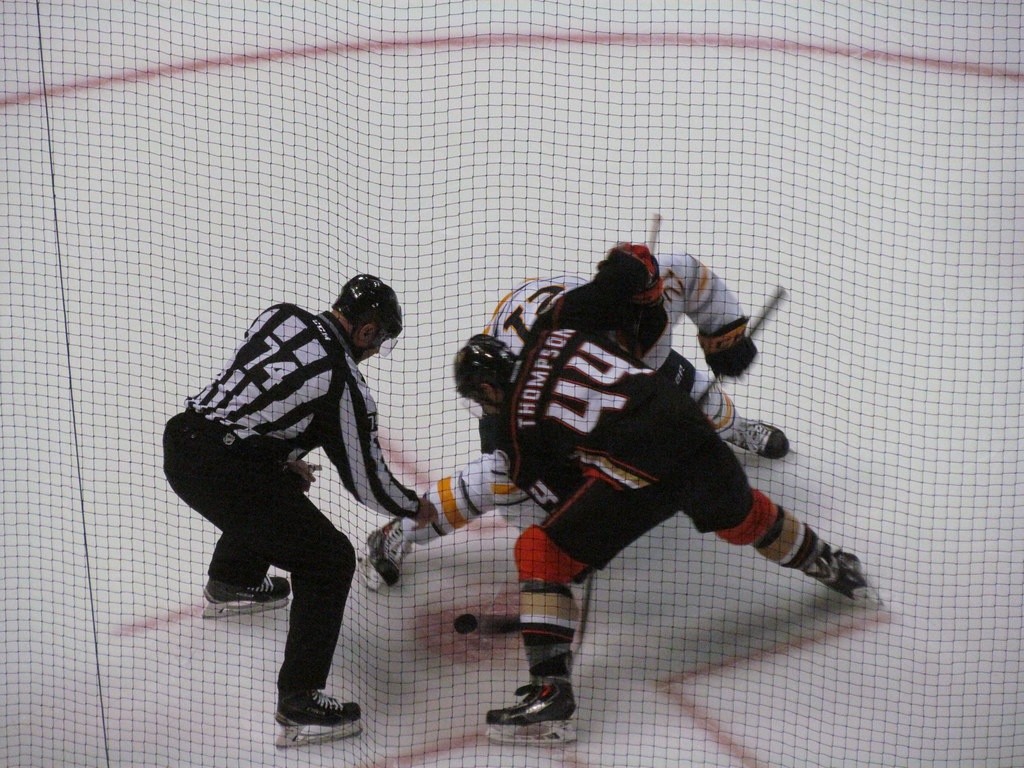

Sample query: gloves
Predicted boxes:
[696,316,758,378]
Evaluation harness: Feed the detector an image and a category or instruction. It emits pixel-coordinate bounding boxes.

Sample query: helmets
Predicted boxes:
[331,274,402,356]
[454,334,515,398]
[608,241,664,306]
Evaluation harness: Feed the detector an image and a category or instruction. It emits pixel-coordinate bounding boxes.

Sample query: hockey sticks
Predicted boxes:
[468,209,785,698]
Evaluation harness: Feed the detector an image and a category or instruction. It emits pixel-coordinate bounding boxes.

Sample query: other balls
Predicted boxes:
[454,613,477,634]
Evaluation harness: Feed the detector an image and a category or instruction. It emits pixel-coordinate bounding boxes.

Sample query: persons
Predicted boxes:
[455,244,881,748]
[357,254,789,588]
[164,273,438,748]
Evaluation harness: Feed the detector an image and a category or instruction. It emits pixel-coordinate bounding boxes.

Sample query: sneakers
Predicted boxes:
[485,651,576,747]
[802,543,884,610]
[201,576,291,618]
[724,417,789,459]
[355,517,413,592]
[272,688,363,747]
